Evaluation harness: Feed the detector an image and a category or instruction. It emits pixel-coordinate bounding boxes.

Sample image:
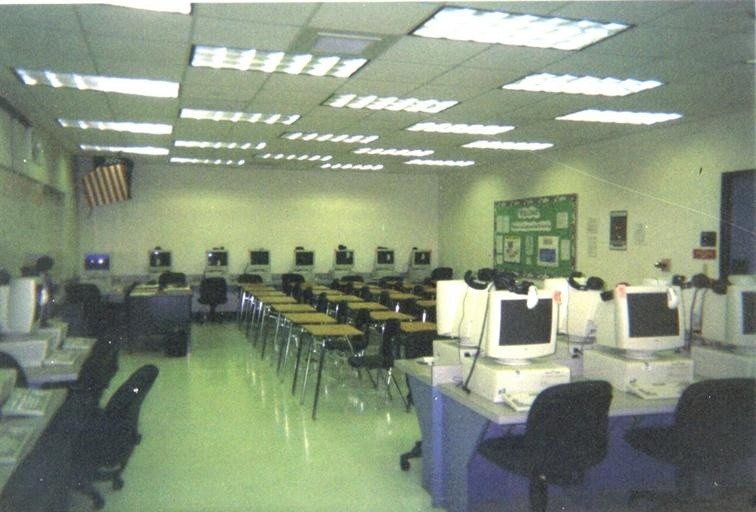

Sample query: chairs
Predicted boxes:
[197,276,229,324]
[236,266,453,421]
[69,272,191,356]
[2,332,160,512]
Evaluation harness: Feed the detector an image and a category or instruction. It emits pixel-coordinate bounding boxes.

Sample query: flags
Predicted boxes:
[81,153,129,209]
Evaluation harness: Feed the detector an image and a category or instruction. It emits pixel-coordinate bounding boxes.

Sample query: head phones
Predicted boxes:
[463,266,539,309]
[569,270,631,302]
[672,264,747,294]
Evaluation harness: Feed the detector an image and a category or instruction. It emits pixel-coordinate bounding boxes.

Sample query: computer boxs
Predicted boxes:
[148,272,165,284]
[463,356,571,405]
[431,339,484,367]
[80,276,112,290]
[582,346,756,393]
[331,269,432,281]
[205,269,316,282]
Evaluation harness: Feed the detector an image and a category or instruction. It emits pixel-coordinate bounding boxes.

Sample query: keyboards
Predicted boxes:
[502,391,548,414]
[416,355,434,366]
[626,377,698,401]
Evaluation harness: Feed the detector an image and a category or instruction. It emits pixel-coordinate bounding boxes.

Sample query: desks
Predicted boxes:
[393,339,752,512]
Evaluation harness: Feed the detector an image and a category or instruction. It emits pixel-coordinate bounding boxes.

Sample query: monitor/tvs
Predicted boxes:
[435,278,500,347]
[294,249,317,271]
[593,283,686,359]
[375,249,396,271]
[247,247,272,272]
[646,278,756,355]
[0,274,99,463]
[206,249,229,271]
[471,285,559,366]
[408,248,432,268]
[84,254,111,275]
[545,273,613,343]
[148,249,173,272]
[334,247,355,269]
